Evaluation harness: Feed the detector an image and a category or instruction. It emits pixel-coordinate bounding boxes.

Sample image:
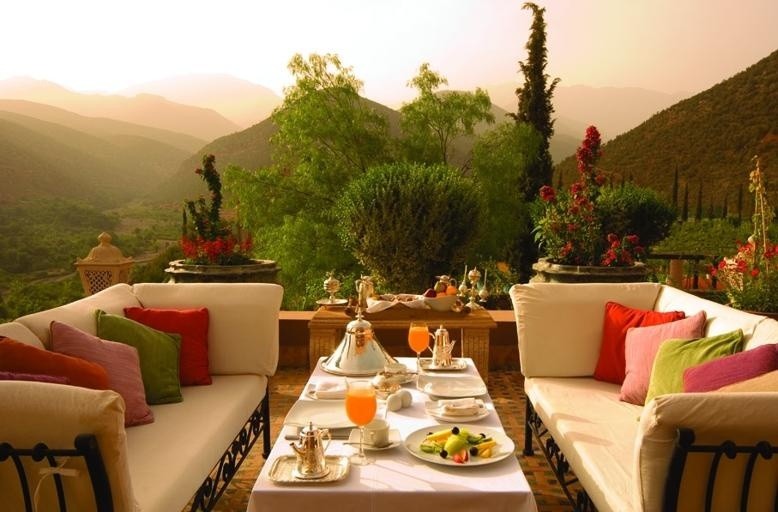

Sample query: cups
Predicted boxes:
[363,418,387,448]
[383,363,411,381]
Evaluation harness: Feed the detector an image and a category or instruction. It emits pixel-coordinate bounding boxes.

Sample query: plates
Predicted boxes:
[425,400,488,423]
[314,299,348,305]
[305,389,345,402]
[404,423,515,466]
[416,373,489,398]
[346,425,401,453]
[377,371,412,385]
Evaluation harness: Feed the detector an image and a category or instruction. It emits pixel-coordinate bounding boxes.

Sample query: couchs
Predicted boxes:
[1,279,283,512]
[511,280,775,511]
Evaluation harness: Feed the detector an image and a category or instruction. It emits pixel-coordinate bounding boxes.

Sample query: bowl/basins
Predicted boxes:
[423,295,459,312]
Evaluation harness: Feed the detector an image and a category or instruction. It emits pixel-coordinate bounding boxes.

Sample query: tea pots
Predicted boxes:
[289,421,332,479]
[426,324,457,367]
[355,276,373,308]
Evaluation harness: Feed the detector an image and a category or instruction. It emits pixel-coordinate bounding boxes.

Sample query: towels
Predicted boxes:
[436,397,486,418]
[314,377,348,400]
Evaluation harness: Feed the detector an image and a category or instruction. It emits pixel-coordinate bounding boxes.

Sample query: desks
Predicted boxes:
[305,304,497,387]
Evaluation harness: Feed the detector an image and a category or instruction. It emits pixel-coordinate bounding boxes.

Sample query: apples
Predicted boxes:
[424,289,436,297]
[434,281,446,291]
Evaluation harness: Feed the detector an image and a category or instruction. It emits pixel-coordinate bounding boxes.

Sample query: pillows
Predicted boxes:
[593,298,684,384]
[94,306,183,406]
[0,334,109,392]
[637,329,743,422]
[0,370,70,388]
[713,369,776,395]
[122,304,212,386]
[678,343,777,391]
[49,318,154,430]
[620,310,706,406]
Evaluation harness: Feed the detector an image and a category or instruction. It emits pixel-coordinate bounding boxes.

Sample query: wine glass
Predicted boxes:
[343,376,379,466]
[408,320,428,376]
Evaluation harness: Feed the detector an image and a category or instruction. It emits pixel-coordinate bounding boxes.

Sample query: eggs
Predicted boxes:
[387,394,402,411]
[396,390,412,407]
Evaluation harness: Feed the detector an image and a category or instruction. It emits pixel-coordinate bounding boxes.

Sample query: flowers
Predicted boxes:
[532,124,646,269]
[706,235,777,312]
[175,152,259,264]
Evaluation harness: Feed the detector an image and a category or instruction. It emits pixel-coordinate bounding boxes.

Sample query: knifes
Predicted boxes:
[285,433,349,440]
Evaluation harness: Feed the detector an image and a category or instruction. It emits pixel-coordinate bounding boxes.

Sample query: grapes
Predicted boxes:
[470,447,477,455]
[452,427,459,434]
[440,450,448,458]
